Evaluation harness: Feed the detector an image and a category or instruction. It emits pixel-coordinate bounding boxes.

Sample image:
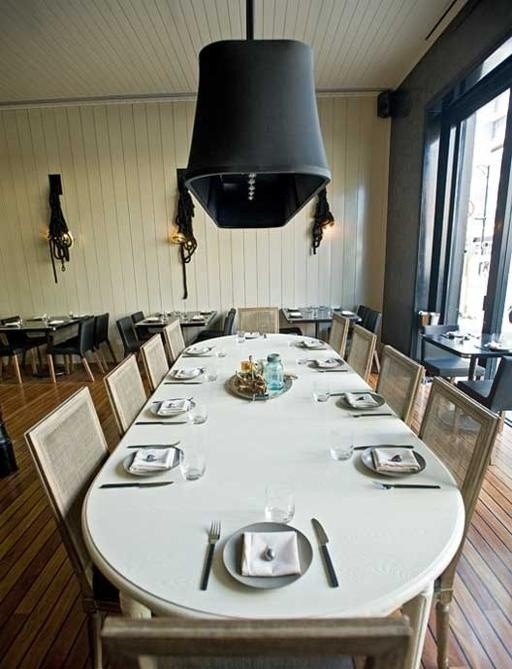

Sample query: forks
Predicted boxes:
[369,481,440,491]
[200,520,222,590]
[127,441,180,449]
[152,397,194,403]
[348,413,392,418]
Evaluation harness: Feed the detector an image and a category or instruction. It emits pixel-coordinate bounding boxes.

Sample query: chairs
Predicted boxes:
[0,315,42,381]
[237,307,280,334]
[98,616,414,669]
[190,308,236,344]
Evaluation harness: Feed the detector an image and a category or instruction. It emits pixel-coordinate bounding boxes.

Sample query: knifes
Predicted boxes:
[164,381,202,385]
[355,444,414,451]
[330,392,370,396]
[312,519,339,588]
[182,355,213,357]
[99,480,174,488]
[317,369,349,373]
[135,420,188,425]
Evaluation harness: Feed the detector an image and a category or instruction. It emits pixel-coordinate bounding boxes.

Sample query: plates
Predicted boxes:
[227,373,296,401]
[307,357,346,369]
[336,393,385,411]
[222,522,314,589]
[168,368,204,379]
[184,347,212,354]
[150,400,195,417]
[244,331,261,341]
[354,450,427,480]
[143,310,212,323]
[288,306,354,317]
[123,446,184,476]
[5,313,85,326]
[441,330,512,351]
[295,337,325,348]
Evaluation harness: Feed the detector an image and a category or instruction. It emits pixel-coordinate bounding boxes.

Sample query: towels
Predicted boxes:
[314,357,341,368]
[186,346,211,354]
[240,530,302,577]
[303,339,322,346]
[175,364,201,379]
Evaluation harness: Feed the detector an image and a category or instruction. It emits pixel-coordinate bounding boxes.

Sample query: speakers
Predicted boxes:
[376,89,394,118]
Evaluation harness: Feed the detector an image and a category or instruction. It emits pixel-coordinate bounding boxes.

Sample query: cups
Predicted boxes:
[178,446,207,481]
[206,367,219,383]
[296,356,307,367]
[263,482,295,526]
[189,401,208,424]
[214,348,228,360]
[236,330,246,344]
[264,353,285,393]
[239,360,250,374]
[313,383,331,402]
[329,433,354,461]
